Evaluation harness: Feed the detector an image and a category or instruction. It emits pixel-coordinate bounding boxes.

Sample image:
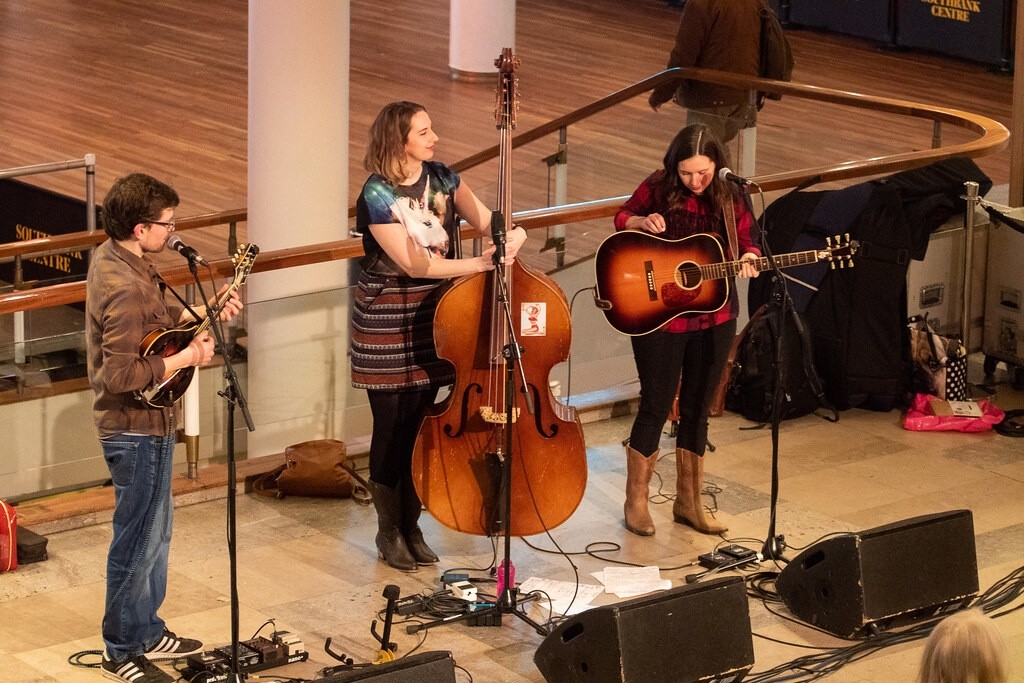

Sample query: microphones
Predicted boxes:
[166,235,209,267]
[719,167,759,189]
[490,210,507,279]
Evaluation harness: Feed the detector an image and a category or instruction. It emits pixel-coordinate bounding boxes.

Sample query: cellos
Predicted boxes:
[411,46,587,577]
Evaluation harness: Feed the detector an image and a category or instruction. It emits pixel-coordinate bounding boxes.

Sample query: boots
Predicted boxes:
[672,447,729,534]
[624,442,660,536]
[368,476,419,573]
[399,478,440,565]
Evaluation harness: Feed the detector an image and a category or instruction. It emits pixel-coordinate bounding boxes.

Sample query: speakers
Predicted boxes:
[533,575,755,683]
[774,510,981,640]
[309,650,456,683]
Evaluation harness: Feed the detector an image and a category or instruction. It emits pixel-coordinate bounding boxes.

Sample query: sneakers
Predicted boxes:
[144,627,204,663]
[101,647,177,683]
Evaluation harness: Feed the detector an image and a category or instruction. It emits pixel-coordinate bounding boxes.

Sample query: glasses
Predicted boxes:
[138,219,176,233]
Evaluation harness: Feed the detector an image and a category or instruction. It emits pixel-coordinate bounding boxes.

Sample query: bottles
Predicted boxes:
[497,560,515,599]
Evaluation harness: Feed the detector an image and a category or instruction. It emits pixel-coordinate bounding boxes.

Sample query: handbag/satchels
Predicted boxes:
[908,314,967,401]
[0,499,18,573]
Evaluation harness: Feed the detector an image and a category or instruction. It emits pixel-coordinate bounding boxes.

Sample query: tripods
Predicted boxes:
[686,182,827,585]
[406,253,549,637]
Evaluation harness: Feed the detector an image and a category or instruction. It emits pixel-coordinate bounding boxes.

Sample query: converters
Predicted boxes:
[395,593,425,617]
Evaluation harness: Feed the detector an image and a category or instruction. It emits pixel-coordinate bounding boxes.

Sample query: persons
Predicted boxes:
[614,123,760,535]
[349,100,527,574]
[648,0,768,170]
[83,172,243,683]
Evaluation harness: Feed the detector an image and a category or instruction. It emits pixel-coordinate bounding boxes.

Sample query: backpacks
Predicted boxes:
[250,438,373,504]
[723,301,841,431]
[754,0,794,113]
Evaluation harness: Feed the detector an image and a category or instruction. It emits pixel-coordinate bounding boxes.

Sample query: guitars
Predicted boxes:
[134,242,260,408]
[594,229,859,335]
